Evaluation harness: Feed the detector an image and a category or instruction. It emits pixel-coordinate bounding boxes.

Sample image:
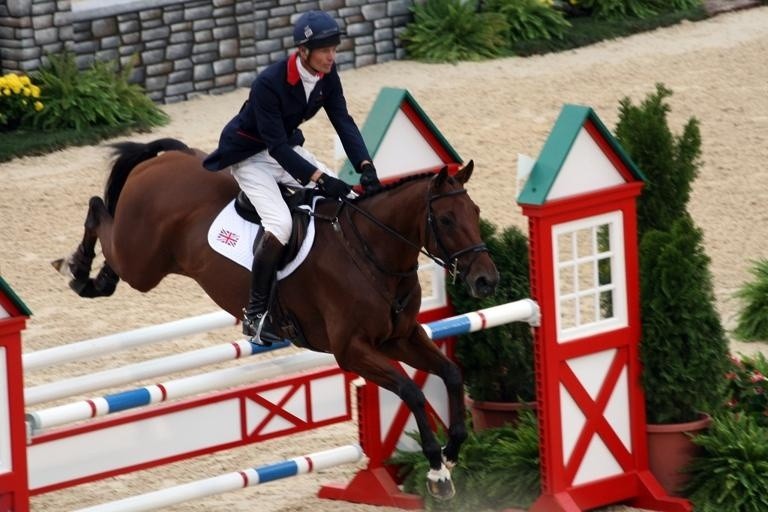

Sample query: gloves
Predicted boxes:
[360,163,381,190]
[316,173,353,198]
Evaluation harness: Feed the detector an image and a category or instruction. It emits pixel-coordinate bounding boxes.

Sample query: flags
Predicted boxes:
[217,229,239,247]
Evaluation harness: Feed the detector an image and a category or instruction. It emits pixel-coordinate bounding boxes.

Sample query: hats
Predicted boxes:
[285,10,347,49]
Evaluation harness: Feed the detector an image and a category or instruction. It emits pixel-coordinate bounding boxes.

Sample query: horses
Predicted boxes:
[48,135,504,504]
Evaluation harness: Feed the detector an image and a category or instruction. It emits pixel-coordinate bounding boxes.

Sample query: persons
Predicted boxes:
[203,10,380,343]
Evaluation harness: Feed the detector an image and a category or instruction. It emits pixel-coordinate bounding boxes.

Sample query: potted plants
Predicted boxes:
[596,78,732,496]
[452,213,541,434]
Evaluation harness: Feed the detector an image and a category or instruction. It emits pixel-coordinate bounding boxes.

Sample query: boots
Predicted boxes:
[242,231,297,340]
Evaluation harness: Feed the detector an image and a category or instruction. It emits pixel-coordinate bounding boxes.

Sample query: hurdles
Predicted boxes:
[1,87,692,512]
